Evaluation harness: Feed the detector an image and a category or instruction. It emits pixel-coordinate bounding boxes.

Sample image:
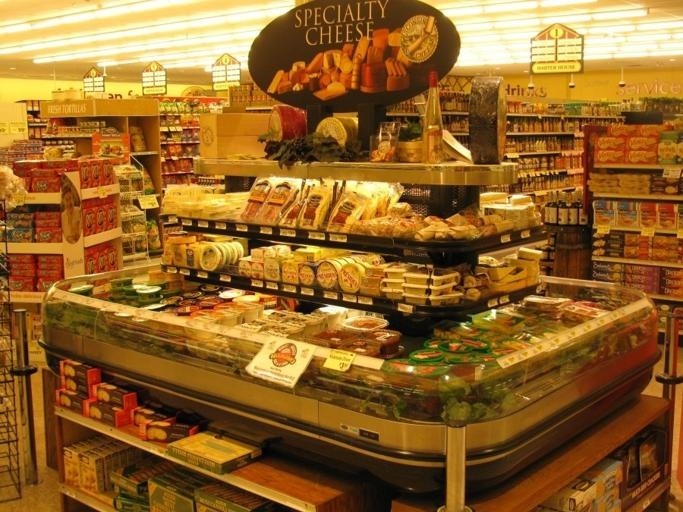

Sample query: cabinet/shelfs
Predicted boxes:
[39,262,662,508]
[113,153,150,264]
[137,97,232,250]
[159,156,551,319]
[0,144,44,169]
[0,184,122,362]
[39,99,164,256]
[591,163,683,335]
[54,393,672,511]
[16,99,48,139]
[539,223,591,276]
[245,106,626,218]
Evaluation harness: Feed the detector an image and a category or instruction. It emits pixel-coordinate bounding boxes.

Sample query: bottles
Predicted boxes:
[558,192,569,225]
[544,191,550,224]
[549,191,557,224]
[569,192,578,224]
[422,70,445,164]
[579,192,588,224]
